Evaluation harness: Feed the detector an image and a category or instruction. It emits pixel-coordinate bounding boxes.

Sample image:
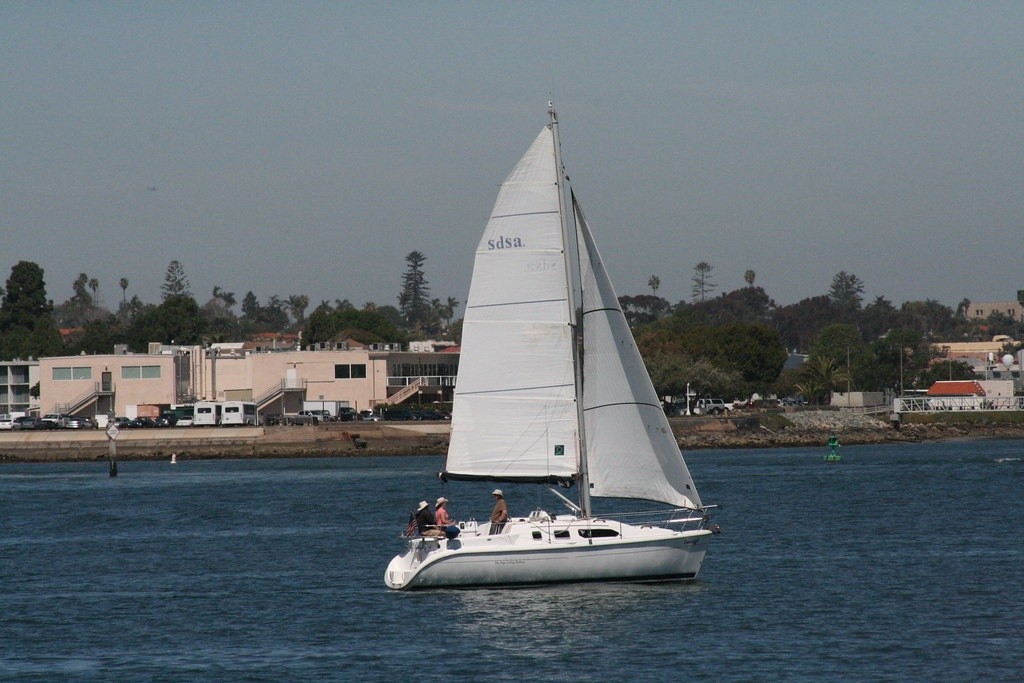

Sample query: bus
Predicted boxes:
[194,402,221,426]
[221,401,259,427]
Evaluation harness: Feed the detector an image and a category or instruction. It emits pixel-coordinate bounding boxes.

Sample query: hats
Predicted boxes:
[491,489,504,497]
[434,497,448,508]
[417,500,429,511]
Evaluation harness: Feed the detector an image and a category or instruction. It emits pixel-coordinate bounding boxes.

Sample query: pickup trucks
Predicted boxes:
[265,410,337,426]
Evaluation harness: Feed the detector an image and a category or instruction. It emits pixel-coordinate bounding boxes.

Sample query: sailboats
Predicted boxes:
[384,99,722,590]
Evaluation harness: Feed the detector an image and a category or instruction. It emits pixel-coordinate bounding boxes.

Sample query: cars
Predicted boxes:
[658,395,809,416]
[113,417,132,427]
[128,416,153,428]
[66,417,94,430]
[176,416,193,427]
[359,406,451,422]
[0,420,21,431]
[21,418,40,430]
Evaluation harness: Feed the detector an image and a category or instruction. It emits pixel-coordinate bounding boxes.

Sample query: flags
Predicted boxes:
[407,510,417,537]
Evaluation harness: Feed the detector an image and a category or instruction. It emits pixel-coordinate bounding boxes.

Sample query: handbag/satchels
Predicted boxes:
[445,526,460,540]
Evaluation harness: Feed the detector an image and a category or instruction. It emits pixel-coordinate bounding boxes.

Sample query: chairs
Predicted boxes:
[409,511,445,538]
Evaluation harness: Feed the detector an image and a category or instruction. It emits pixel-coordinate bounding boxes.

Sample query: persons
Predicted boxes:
[434,497,461,540]
[489,487,508,535]
[417,500,446,547]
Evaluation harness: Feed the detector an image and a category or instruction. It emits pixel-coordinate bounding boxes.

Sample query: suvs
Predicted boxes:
[41,414,64,430]
[155,415,175,428]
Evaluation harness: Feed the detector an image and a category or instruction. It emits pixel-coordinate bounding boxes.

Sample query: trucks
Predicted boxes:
[303,401,363,422]
